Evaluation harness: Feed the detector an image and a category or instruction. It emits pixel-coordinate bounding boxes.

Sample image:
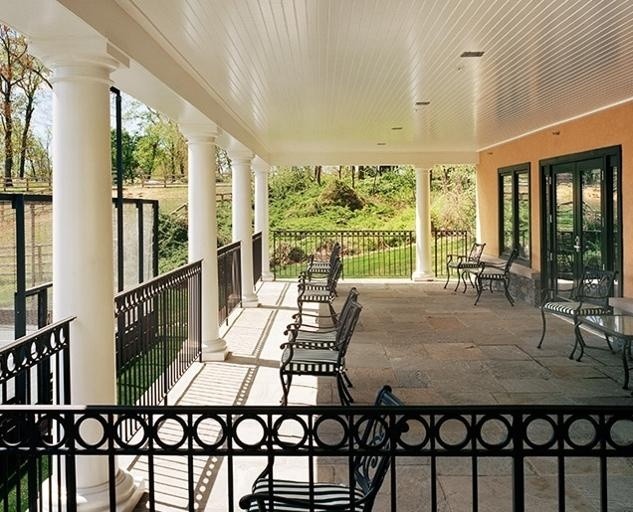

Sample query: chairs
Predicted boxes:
[237,384,406,511]
[291,241,344,331]
[537,262,619,360]
[443,243,488,292]
[279,284,365,407]
[462,247,520,305]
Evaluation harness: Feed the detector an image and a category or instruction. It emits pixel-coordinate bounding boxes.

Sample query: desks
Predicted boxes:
[574,314,633,393]
